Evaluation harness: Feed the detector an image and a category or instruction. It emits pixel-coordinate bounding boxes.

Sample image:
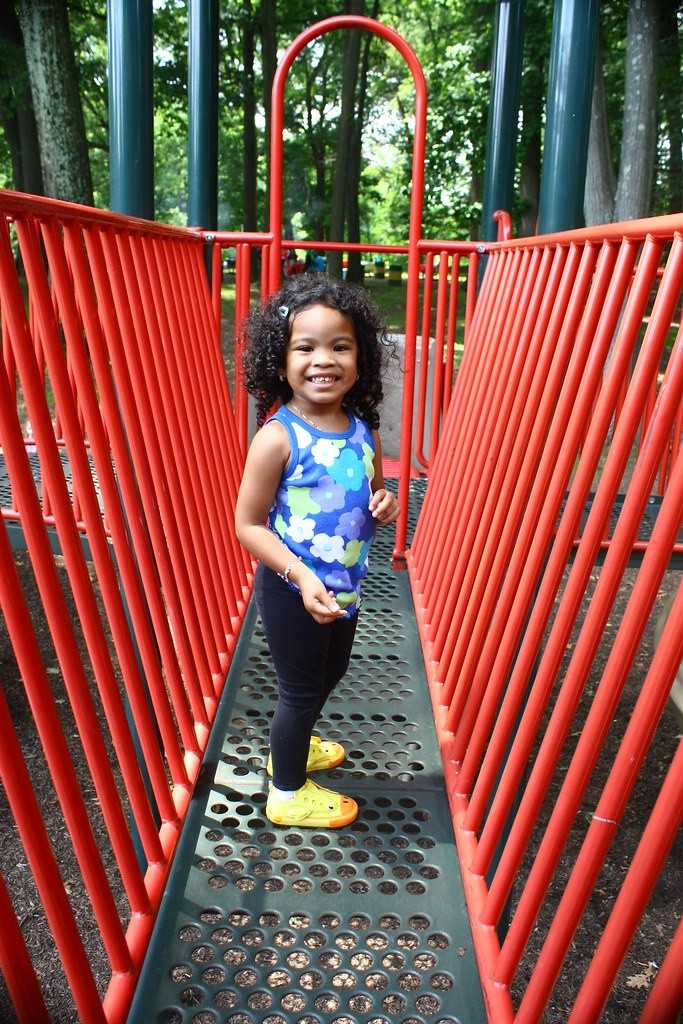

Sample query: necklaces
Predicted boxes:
[286,398,324,431]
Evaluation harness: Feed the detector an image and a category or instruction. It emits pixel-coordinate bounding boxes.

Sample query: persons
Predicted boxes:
[224,247,383,278]
[229,272,405,828]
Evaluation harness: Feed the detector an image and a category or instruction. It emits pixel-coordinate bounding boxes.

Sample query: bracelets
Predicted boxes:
[277,555,303,584]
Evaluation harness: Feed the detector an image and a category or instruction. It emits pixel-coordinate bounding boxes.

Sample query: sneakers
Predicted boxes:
[267,736,345,776]
[266,778,358,827]
